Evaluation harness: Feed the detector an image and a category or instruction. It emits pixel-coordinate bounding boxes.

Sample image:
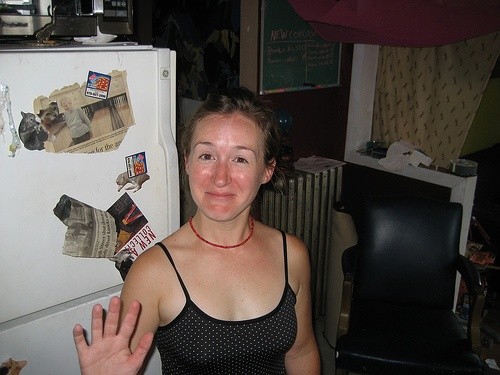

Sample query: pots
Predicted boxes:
[447,159,478,177]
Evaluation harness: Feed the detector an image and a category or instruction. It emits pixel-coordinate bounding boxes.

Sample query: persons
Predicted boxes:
[58,96,94,145]
[71,85,321,375]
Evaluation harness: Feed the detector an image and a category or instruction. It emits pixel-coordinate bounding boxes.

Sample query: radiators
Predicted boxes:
[260,158,343,319]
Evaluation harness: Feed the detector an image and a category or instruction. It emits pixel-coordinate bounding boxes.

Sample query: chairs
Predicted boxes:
[330,194,484,375]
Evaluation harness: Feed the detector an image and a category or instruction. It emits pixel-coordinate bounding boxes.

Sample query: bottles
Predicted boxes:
[456,294,469,335]
[470,296,473,305]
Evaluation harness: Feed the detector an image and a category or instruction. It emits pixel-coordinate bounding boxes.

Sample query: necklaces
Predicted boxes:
[188,215,256,249]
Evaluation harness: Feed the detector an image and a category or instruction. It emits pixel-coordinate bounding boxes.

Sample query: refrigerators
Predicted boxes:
[0,45,180,375]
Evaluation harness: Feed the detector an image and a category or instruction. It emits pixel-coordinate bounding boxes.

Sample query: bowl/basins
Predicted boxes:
[73,25,117,44]
